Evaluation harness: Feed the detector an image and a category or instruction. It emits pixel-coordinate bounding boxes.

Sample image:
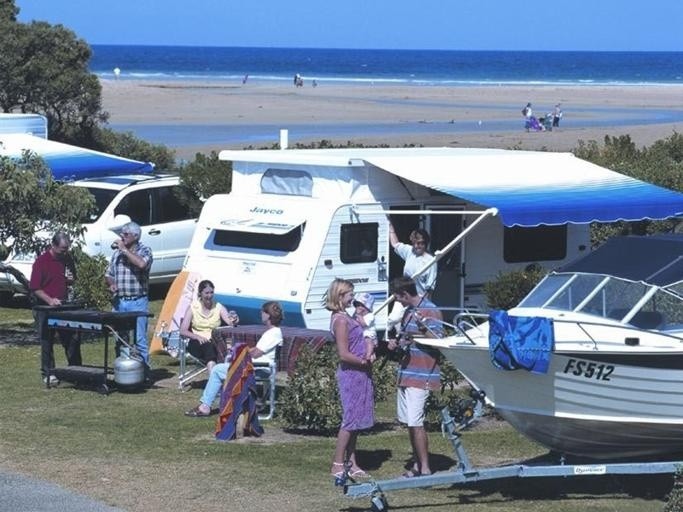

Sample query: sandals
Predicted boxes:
[330,462,350,478]
[348,470,370,480]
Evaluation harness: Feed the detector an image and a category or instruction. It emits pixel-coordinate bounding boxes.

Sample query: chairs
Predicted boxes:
[178,317,207,390]
[222,343,278,421]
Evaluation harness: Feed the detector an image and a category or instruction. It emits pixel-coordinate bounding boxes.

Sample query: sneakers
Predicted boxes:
[144,375,154,387]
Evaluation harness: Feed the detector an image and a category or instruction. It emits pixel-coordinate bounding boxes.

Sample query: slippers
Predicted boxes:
[184,405,210,416]
[402,470,421,478]
[419,474,430,477]
[42,375,57,384]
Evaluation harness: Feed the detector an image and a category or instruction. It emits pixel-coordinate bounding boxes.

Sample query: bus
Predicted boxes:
[180,149,593,348]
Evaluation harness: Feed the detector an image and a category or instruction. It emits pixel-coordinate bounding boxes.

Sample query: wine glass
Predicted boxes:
[228,311,237,329]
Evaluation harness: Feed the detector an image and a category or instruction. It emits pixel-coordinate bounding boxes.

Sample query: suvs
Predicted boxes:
[0,174,203,304]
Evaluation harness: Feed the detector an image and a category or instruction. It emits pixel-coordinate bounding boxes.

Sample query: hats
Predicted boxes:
[351,292,374,312]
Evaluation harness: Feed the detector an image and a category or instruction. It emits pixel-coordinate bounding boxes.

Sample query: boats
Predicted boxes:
[413,236,683,454]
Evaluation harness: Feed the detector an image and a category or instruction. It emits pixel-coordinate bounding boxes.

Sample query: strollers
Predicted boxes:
[519,113,567,136]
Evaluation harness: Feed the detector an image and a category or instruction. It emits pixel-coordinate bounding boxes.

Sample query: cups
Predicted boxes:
[111,242,119,249]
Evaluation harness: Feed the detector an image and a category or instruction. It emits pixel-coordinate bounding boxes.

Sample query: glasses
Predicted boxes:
[119,233,132,236]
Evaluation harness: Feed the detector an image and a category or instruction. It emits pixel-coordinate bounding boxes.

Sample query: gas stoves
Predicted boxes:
[33,302,154,322]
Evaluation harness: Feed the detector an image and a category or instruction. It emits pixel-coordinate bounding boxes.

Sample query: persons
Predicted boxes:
[181,280,239,374]
[31,233,83,385]
[524,102,533,132]
[389,221,438,327]
[353,292,377,363]
[185,302,284,416]
[389,277,439,479]
[324,279,374,479]
[550,101,561,128]
[105,222,152,367]
